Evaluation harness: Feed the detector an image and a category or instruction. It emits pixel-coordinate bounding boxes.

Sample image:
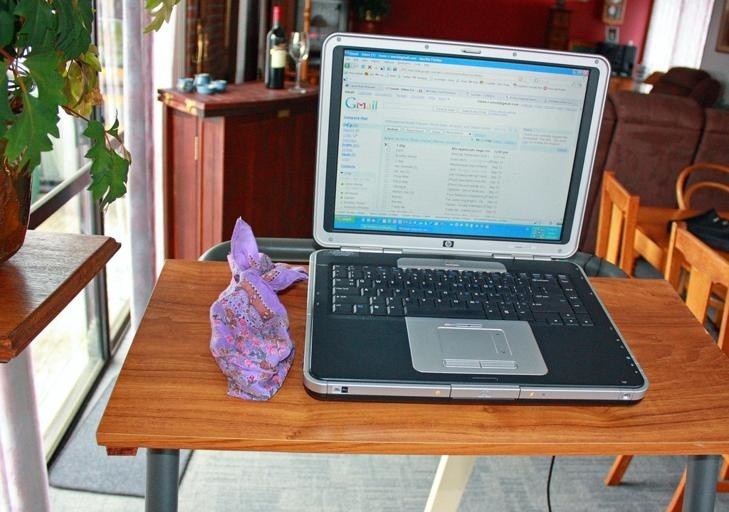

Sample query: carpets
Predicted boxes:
[48,375,194,498]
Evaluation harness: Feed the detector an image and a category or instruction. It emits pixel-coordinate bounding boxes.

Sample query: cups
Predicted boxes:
[177,77,194,93]
[290,31,310,95]
[194,73,209,91]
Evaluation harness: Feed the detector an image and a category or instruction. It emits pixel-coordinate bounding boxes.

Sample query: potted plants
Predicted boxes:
[0,0,181,265]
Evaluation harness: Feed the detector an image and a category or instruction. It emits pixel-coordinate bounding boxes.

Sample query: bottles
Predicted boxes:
[265,6,286,89]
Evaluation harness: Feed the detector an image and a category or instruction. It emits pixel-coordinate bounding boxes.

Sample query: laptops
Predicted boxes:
[302,31,649,404]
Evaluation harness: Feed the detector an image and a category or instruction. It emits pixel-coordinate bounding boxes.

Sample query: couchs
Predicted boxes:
[678,108,729,212]
[645,67,722,108]
[580,91,704,253]
[579,90,616,250]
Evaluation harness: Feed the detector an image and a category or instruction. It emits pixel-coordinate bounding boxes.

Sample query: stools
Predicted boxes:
[0,230,121,511]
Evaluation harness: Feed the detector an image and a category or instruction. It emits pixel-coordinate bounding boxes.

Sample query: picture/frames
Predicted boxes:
[602,0,626,24]
[604,25,619,45]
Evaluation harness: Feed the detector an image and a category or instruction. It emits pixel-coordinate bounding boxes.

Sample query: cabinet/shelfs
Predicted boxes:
[157,84,320,260]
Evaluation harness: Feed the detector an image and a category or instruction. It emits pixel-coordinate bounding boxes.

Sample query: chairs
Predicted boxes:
[676,163,729,212]
[593,168,666,277]
[605,222,729,511]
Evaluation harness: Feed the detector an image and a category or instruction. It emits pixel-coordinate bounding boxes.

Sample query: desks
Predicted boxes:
[95,237,728,511]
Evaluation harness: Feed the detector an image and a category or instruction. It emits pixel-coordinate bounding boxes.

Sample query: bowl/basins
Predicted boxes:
[210,80,226,91]
[197,84,215,94]
